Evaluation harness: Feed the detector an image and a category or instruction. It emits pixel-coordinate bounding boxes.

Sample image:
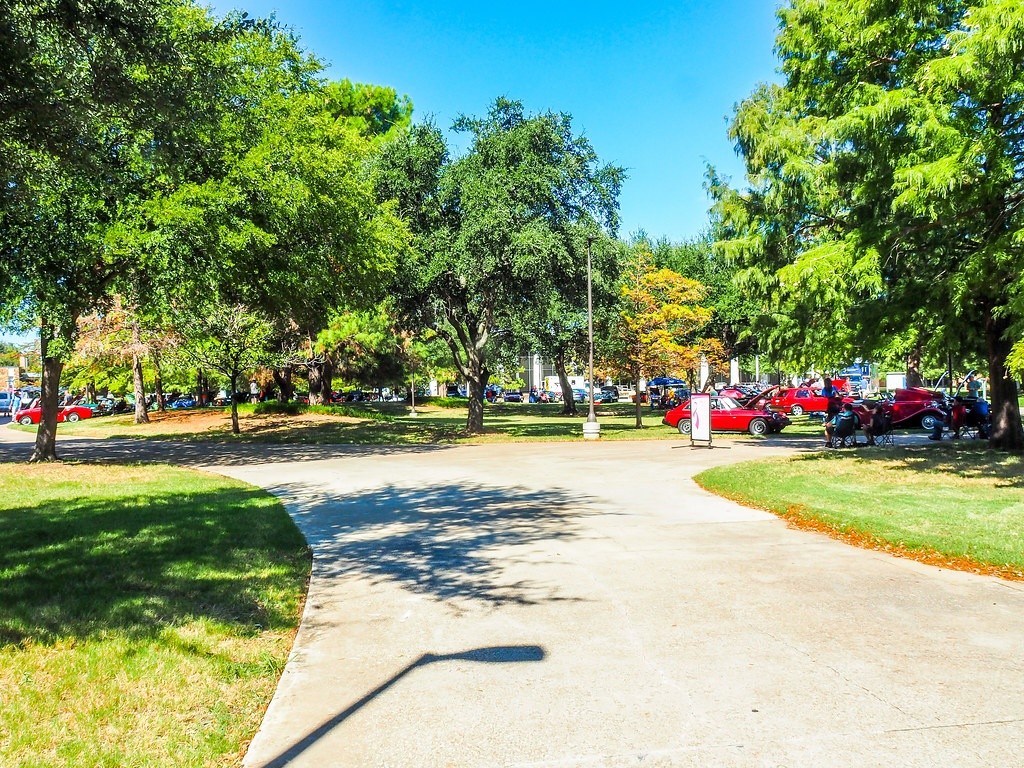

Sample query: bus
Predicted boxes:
[150,393,172,402]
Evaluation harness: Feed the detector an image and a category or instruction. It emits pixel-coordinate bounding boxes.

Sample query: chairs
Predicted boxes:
[961,402,987,440]
[866,414,894,448]
[942,412,970,439]
[826,417,857,448]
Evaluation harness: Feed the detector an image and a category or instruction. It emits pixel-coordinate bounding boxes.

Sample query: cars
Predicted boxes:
[529,388,556,403]
[662,385,792,434]
[594,386,619,403]
[947,396,993,423]
[769,387,855,416]
[504,392,524,403]
[572,389,587,403]
[843,387,952,430]
[633,391,655,403]
[15,396,92,425]
[661,388,702,408]
[170,398,196,408]
[446,391,460,397]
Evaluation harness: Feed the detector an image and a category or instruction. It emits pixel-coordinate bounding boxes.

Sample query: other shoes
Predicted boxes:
[825,441,833,448]
[953,433,960,439]
[865,437,875,445]
[928,433,941,441]
[839,441,846,447]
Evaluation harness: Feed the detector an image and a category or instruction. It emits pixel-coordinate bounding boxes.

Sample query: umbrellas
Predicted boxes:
[647,377,685,394]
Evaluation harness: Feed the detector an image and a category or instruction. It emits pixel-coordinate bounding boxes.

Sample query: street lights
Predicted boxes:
[905,352,911,374]
[582,235,602,441]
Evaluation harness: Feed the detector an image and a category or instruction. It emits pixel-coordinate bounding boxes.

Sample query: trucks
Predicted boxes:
[544,376,595,393]
[838,362,880,399]
[0,392,39,412]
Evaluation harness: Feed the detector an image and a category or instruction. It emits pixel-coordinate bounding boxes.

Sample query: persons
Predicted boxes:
[491,384,497,404]
[795,377,868,399]
[928,396,965,441]
[967,375,982,397]
[147,395,153,407]
[864,406,886,445]
[705,386,718,396]
[63,390,73,406]
[250,380,267,404]
[650,386,675,410]
[969,398,988,419]
[824,404,858,448]
[9,392,22,422]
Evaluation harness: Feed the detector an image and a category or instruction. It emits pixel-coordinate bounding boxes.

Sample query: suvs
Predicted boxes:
[717,386,761,406]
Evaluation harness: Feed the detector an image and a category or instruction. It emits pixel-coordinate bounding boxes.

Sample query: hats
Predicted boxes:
[951,396,963,404]
